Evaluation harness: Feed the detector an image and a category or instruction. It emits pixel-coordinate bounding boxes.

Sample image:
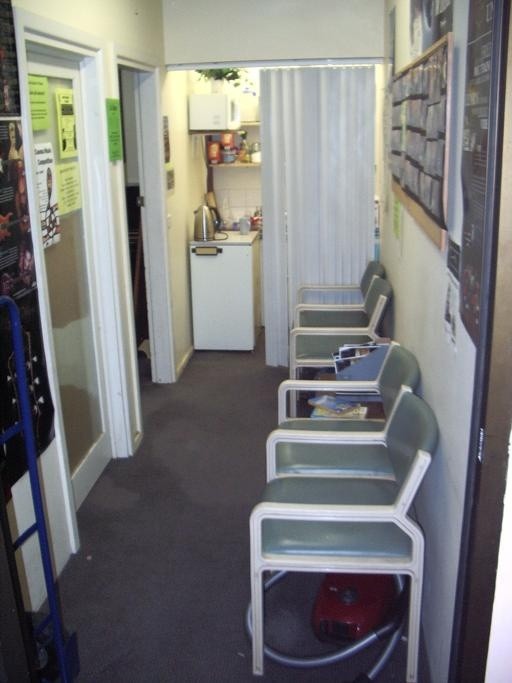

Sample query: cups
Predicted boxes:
[240,217,251,234]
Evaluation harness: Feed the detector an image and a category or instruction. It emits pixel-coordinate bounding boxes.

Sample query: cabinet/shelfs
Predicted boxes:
[187,230,261,354]
[207,121,261,167]
[119,67,139,186]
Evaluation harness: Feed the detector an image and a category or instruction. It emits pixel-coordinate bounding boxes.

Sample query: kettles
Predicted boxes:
[193,204,221,241]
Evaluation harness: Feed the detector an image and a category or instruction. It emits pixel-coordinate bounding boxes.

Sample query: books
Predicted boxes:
[308,390,362,415]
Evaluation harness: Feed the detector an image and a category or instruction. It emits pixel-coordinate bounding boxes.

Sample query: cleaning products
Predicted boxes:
[237,130,252,164]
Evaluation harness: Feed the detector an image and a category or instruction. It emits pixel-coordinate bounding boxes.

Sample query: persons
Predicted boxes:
[15,120,23,159]
[7,120,21,160]
